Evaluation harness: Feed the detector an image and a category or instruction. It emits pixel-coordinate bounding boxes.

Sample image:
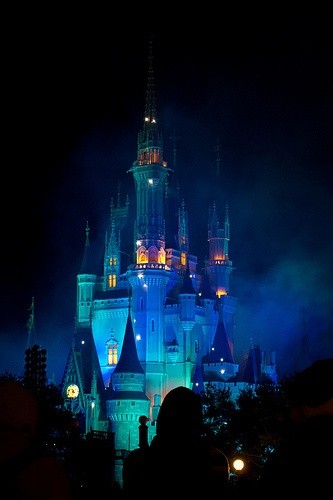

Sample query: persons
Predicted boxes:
[122,386,230,500]
[0,380,71,500]
